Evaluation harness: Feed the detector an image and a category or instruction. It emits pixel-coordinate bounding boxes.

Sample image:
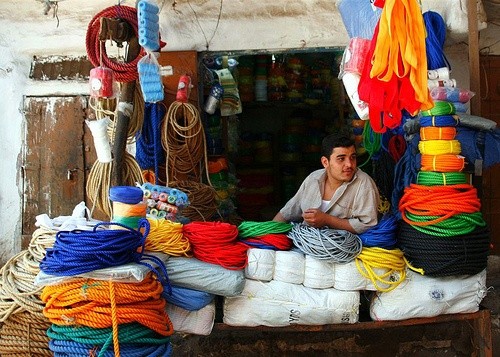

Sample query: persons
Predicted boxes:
[272,132,380,234]
